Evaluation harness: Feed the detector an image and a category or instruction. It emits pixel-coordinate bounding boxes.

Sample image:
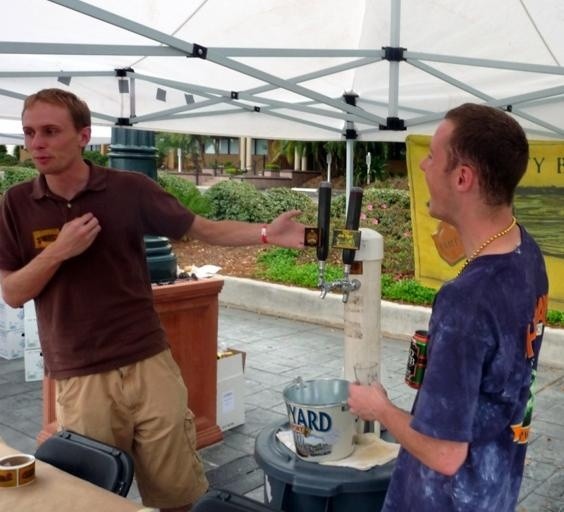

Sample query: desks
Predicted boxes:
[0,439,161,512]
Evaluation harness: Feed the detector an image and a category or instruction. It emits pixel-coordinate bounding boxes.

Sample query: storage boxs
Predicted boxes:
[0,298,45,382]
[214,348,246,432]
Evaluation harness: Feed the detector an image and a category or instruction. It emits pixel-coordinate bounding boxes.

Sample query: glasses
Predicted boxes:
[179,272,197,281]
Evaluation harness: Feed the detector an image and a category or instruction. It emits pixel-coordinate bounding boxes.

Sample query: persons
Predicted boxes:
[2,88,317,512]
[347,99,550,512]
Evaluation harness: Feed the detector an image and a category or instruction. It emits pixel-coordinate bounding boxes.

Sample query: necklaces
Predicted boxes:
[453,216,519,280]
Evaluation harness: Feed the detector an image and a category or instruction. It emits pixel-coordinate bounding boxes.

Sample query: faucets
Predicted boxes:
[317,260,341,300]
[339,270,361,303]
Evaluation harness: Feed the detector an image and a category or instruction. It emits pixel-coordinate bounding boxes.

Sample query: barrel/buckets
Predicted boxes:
[282,374,358,464]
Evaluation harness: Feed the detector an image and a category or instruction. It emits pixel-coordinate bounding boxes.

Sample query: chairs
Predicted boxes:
[188,489,279,512]
[33,429,134,497]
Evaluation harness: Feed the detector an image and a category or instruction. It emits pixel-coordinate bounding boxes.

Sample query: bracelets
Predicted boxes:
[261,222,271,246]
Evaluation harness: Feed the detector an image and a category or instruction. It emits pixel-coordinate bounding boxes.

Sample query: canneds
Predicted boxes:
[405,330,430,390]
[0,454,35,488]
